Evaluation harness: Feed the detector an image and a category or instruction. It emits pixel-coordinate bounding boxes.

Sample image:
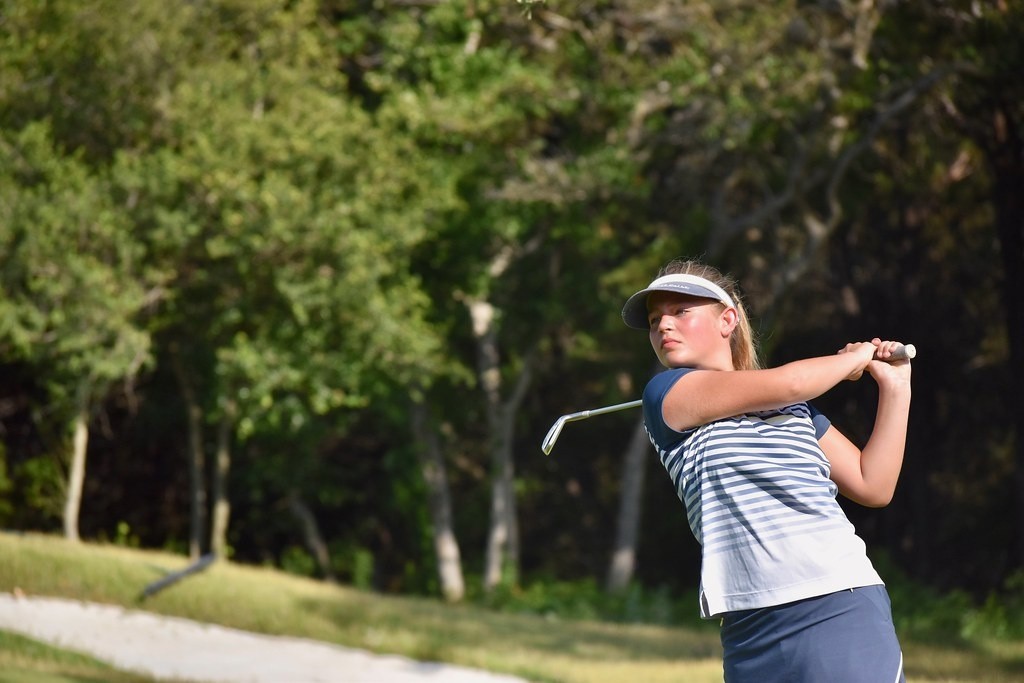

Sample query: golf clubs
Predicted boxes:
[540,339,920,458]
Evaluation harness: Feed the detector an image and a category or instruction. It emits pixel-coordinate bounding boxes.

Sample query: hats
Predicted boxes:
[621,273,741,330]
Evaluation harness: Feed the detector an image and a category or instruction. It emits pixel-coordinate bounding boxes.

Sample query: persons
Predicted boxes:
[642,257,911,683]
